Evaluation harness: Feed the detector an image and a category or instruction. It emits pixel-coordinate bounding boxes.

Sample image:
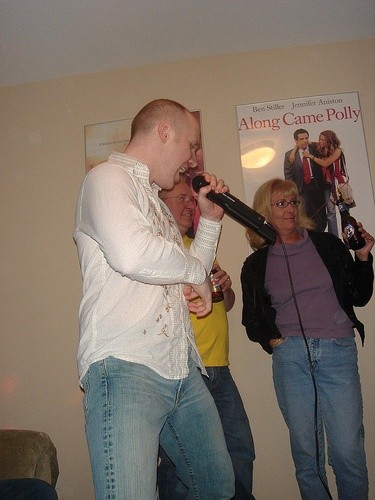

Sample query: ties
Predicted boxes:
[302,157,312,185]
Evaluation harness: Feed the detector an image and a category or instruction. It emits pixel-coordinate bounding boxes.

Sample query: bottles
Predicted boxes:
[209,267,224,303]
[336,199,366,251]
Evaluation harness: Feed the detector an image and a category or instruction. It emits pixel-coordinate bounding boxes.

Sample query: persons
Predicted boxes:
[72,98,257,500]
[240,178,375,500]
[283,128,356,251]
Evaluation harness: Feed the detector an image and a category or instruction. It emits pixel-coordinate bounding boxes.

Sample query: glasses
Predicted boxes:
[165,195,195,203]
[270,199,300,208]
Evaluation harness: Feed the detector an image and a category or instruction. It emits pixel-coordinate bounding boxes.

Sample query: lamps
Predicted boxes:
[241,141,276,169]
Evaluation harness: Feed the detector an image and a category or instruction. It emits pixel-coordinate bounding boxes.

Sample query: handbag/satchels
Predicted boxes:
[330,175,353,205]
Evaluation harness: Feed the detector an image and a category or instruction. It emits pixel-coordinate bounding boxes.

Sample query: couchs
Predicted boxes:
[0,429,59,489]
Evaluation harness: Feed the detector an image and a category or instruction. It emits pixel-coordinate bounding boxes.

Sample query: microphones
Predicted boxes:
[191,175,277,245]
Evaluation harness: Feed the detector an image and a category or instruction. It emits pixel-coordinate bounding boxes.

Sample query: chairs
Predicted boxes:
[0,478,59,500]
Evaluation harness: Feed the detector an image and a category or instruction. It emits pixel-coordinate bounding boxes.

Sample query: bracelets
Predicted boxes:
[313,156,315,160]
[270,339,282,346]
